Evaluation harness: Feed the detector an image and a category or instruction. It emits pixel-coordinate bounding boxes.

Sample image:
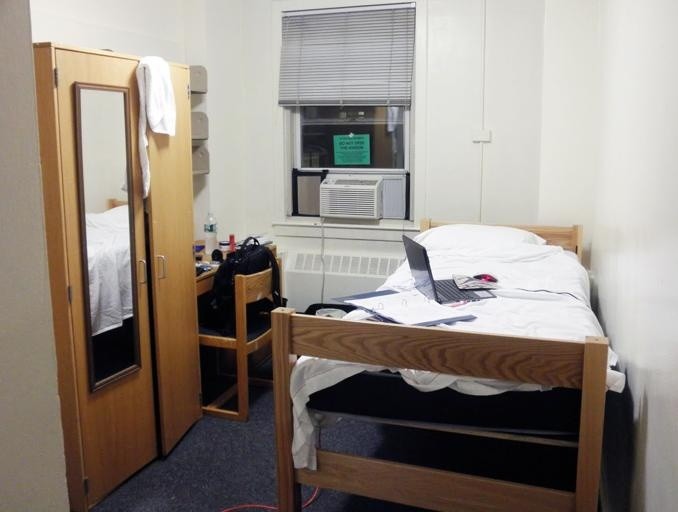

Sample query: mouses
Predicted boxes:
[474,274,498,283]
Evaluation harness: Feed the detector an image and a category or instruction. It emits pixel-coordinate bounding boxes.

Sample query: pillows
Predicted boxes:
[411,223,546,256]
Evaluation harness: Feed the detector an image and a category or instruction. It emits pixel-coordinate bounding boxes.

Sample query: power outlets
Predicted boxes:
[473,129,492,143]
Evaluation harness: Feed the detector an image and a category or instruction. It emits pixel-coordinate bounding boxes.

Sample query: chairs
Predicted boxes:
[199,259,285,420]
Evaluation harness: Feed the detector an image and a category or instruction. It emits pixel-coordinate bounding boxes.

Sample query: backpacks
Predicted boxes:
[206,238,288,338]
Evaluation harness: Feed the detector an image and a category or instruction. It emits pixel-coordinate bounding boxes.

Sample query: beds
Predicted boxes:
[272,224,609,511]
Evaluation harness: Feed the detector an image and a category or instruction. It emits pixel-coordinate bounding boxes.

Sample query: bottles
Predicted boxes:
[204,211,218,255]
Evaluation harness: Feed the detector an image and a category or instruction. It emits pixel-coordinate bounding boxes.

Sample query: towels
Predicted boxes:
[134,56,175,198]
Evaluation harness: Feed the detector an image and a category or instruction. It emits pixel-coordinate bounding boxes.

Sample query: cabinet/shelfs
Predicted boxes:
[34,42,203,507]
[287,109,409,225]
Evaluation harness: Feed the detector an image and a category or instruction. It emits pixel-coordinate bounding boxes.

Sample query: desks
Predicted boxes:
[196,242,280,297]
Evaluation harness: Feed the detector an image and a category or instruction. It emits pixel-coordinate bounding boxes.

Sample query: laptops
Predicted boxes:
[402,234,496,303]
